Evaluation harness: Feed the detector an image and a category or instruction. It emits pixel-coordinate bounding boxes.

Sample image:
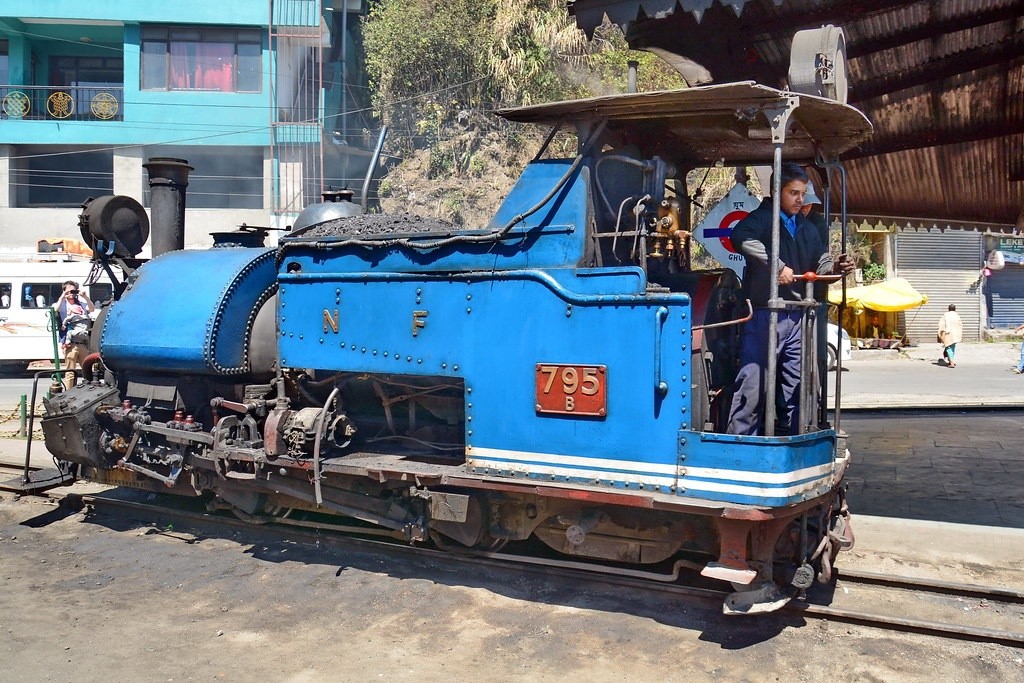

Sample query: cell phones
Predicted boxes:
[71,290,79,294]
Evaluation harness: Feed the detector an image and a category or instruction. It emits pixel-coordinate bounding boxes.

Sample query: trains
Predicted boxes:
[0,23,877,617]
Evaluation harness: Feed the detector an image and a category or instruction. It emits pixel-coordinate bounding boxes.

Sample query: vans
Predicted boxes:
[0,251,126,374]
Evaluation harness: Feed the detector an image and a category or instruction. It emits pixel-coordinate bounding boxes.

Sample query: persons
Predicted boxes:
[725,160,854,435]
[1013,325,1024,373]
[49,280,94,389]
[938,304,962,368]
[865,316,883,338]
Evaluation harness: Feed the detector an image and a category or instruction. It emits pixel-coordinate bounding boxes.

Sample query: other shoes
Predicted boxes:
[947,362,955,368]
[1012,369,1021,374]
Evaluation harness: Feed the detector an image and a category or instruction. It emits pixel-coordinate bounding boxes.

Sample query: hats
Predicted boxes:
[70,304,83,315]
[801,178,822,206]
